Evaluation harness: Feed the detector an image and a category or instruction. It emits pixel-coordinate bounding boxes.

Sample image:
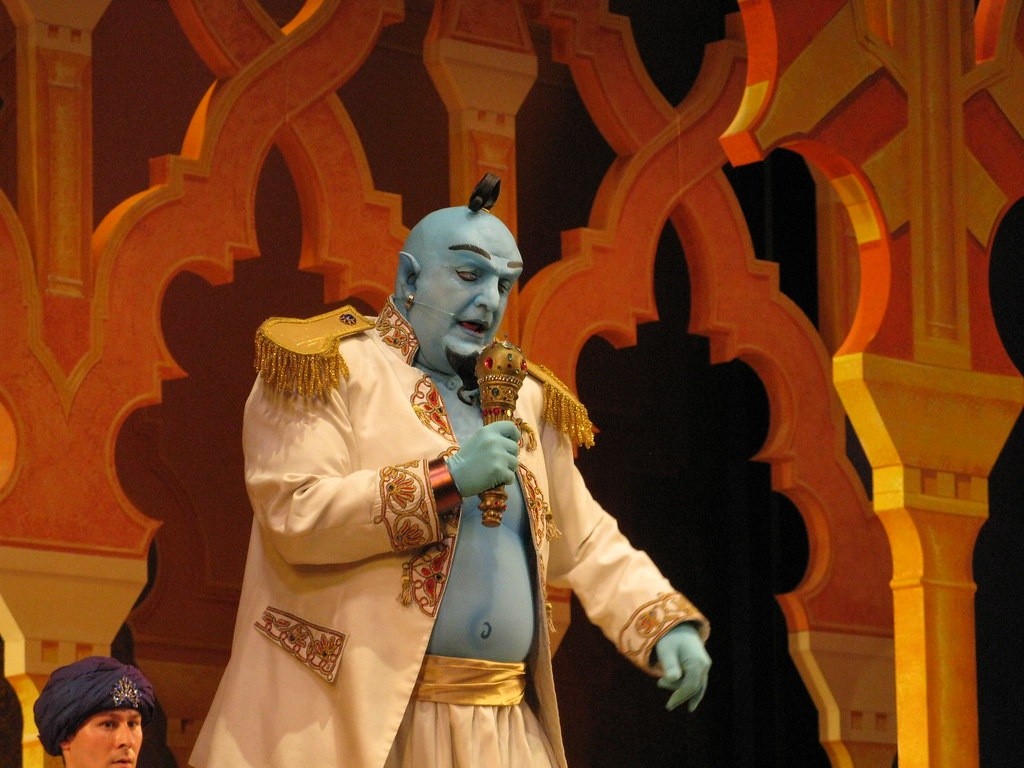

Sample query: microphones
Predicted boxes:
[410,301,455,318]
[476,342,527,528]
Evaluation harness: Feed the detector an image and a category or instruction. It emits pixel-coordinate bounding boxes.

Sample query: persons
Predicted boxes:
[33,656,156,768]
[190,208,712,768]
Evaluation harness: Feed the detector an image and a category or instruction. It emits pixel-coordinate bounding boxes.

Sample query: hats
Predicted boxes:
[32,656,156,755]
